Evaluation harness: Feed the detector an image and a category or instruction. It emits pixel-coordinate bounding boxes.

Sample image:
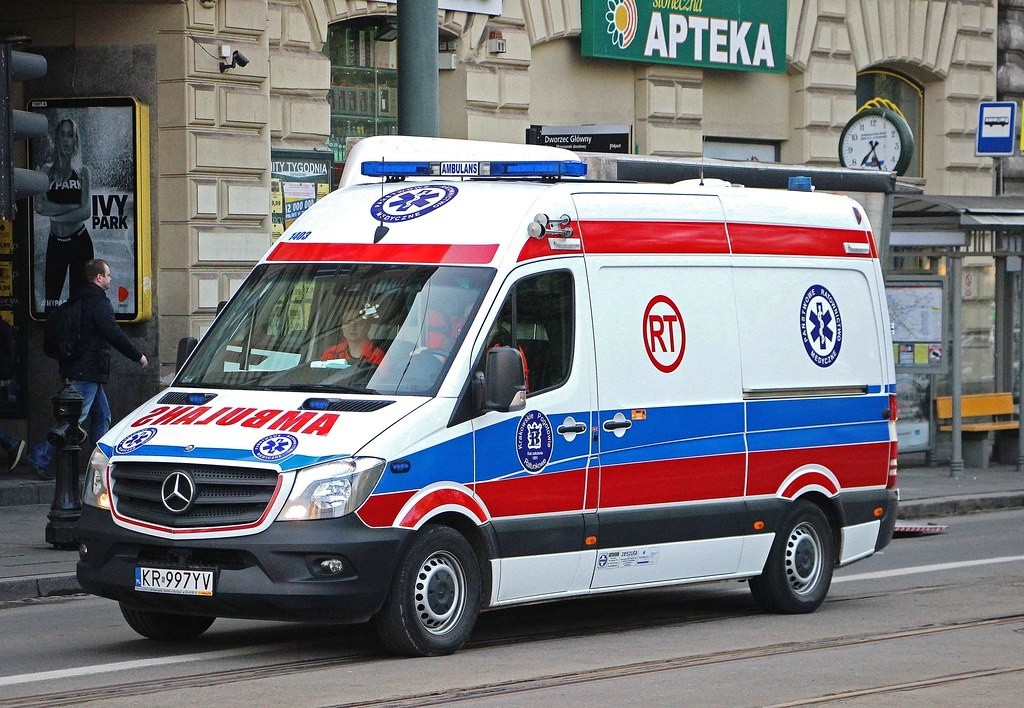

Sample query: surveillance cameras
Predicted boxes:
[233,51,250,67]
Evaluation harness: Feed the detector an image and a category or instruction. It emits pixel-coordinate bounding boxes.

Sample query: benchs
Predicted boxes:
[936,392,1020,469]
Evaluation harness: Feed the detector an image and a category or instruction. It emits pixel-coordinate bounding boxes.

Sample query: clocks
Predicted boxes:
[837,107,914,177]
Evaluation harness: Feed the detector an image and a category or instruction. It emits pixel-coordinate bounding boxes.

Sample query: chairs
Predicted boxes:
[503,291,550,389]
[364,291,408,340]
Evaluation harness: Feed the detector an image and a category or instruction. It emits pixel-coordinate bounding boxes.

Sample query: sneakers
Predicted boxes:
[7,439,25,472]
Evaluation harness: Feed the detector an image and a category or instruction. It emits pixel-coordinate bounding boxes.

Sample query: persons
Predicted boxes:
[27,258,150,481]
[408,294,531,413]
[36,117,96,312]
[314,312,389,374]
[0,313,25,473]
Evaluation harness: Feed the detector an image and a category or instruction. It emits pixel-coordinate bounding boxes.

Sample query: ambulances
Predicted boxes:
[74,155,899,658]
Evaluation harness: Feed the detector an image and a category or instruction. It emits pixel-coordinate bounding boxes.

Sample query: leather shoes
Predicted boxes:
[31,460,55,481]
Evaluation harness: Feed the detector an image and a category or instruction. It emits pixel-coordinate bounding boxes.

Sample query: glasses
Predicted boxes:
[343,320,366,326]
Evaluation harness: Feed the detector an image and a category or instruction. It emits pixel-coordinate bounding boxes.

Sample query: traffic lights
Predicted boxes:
[1,43,50,220]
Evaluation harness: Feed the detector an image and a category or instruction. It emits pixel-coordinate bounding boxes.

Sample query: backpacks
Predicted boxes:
[43,295,83,364]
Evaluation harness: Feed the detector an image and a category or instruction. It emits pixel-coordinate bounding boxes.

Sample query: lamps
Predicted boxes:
[372,17,399,42]
